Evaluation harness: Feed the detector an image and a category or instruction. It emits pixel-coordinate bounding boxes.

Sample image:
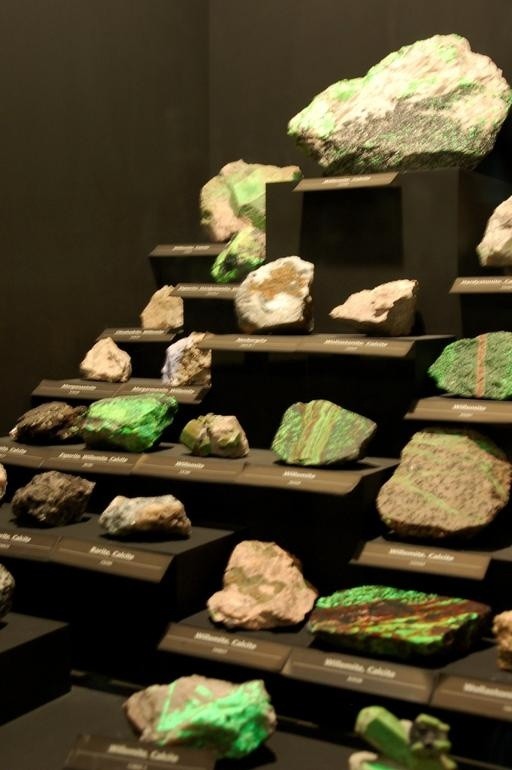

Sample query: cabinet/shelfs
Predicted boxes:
[0,167,512,770]
